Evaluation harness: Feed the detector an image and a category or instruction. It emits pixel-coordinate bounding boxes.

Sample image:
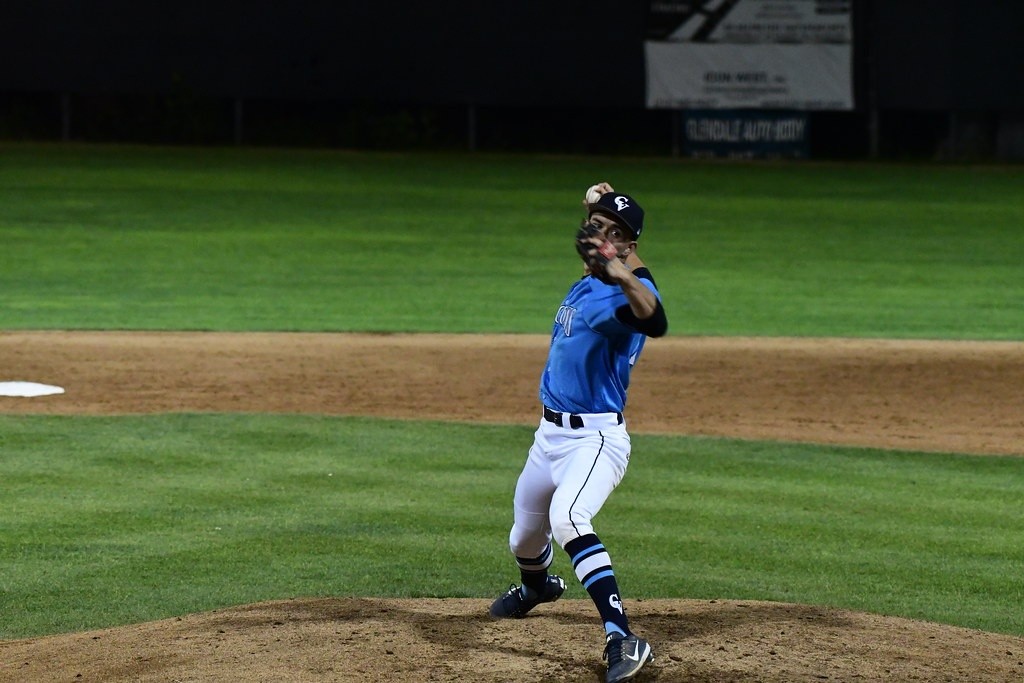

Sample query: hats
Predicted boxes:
[588,192,644,244]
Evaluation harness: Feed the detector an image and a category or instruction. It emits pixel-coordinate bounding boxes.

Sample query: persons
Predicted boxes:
[489,183,669,683]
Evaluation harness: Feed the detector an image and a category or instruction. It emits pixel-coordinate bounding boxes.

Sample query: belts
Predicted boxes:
[542,406,622,430]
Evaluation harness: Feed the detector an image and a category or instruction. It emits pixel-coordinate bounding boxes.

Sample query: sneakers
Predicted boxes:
[489,574,565,618]
[603,634,652,683]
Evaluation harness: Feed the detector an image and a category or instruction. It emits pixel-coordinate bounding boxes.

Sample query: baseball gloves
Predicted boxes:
[575,217,619,287]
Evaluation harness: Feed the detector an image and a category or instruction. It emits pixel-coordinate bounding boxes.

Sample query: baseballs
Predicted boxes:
[586,185,602,203]
